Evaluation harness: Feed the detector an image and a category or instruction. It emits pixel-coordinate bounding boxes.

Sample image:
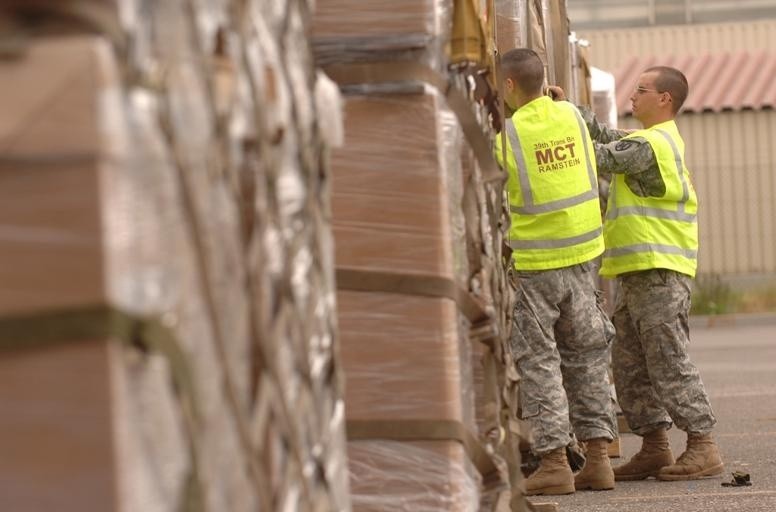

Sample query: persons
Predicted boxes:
[493,49,615,496]
[545,66,725,485]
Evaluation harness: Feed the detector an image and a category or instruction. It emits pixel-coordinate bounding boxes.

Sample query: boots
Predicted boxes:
[611,427,675,480]
[523,447,575,496]
[658,431,725,480]
[574,437,615,491]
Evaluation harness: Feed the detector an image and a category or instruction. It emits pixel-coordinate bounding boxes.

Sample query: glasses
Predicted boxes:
[633,83,672,102]
